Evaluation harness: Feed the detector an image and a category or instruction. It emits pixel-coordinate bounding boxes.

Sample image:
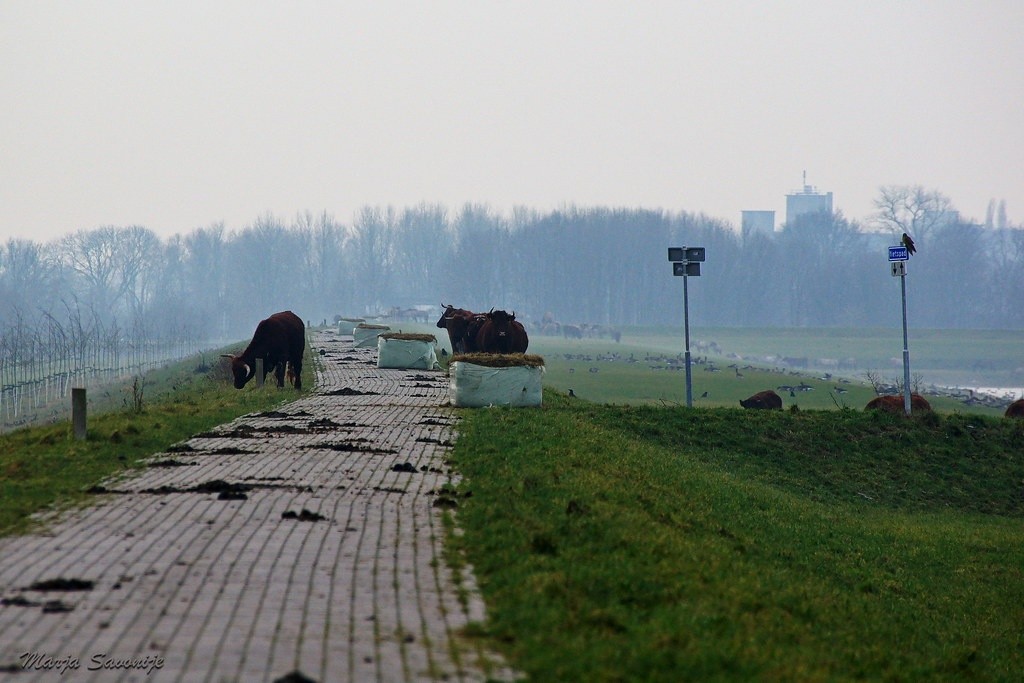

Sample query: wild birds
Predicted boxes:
[838,378,852,383]
[777,382,815,397]
[564,352,832,381]
[442,348,447,356]
[568,389,576,398]
[701,392,708,397]
[903,233,916,256]
[834,386,848,393]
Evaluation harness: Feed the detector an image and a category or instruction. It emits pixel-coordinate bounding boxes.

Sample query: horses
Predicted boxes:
[687,339,910,373]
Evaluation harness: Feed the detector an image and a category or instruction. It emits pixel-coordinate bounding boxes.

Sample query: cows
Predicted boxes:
[437,303,528,355]
[221,311,305,390]
[863,396,931,413]
[740,390,782,410]
[397,302,622,344]
[1005,399,1024,418]
